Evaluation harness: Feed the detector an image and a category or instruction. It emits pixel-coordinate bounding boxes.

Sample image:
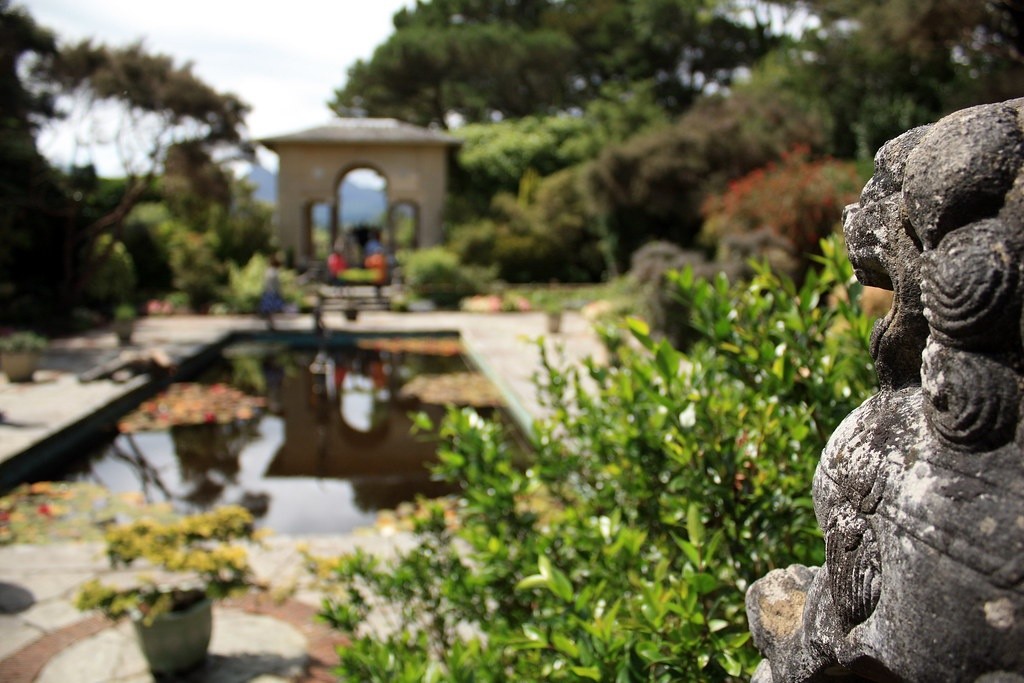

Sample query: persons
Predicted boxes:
[256,258,285,329]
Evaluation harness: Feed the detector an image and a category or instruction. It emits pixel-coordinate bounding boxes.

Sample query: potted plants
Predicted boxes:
[76,500,270,674]
[0,331,49,383]
[408,246,492,311]
[113,303,137,346]
